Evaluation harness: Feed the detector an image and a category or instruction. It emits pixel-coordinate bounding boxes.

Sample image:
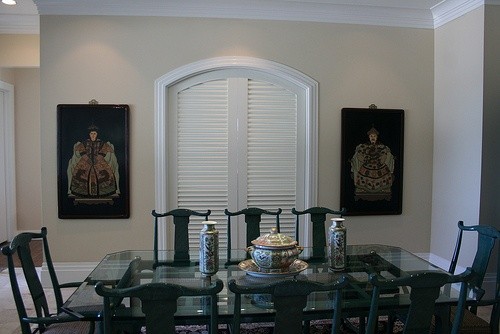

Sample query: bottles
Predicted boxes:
[327,218,347,272]
[199,220,220,275]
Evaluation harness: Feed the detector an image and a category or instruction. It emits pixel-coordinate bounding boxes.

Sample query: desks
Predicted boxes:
[59,244,477,333]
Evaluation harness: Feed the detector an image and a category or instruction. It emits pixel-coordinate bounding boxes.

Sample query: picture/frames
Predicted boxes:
[338,108,406,218]
[56,104,132,219]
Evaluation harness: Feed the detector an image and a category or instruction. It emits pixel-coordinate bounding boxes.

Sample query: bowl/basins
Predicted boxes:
[245,227,304,272]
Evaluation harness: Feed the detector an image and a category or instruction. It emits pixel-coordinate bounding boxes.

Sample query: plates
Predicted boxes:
[237,258,308,278]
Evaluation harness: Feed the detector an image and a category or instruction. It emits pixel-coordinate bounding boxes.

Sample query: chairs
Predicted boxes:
[1,206,500,334]
